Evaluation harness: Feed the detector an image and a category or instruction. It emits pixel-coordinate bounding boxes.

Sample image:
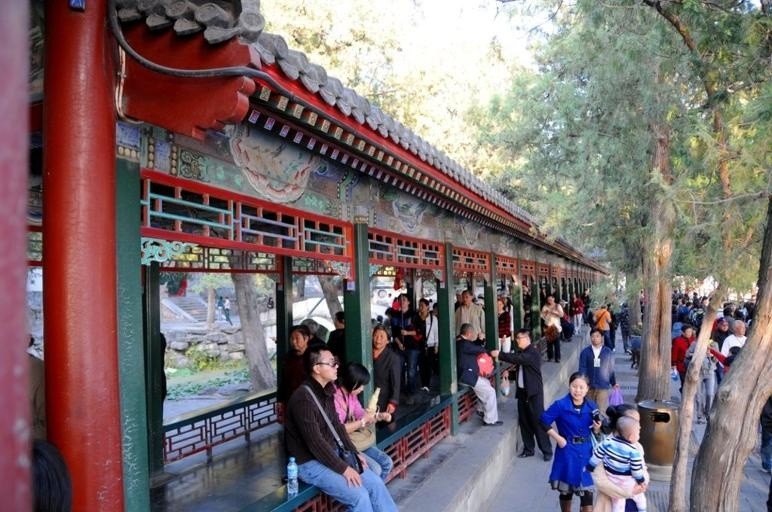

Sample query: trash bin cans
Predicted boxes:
[638,399,678,466]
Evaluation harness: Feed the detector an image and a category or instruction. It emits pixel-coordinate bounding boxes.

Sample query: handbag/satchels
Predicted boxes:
[543,325,560,343]
[591,322,597,330]
[342,450,363,474]
[347,426,376,452]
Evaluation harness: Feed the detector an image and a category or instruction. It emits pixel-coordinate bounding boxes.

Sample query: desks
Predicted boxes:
[631,349,640,368]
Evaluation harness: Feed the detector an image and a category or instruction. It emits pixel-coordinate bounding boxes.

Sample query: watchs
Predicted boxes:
[360,418,365,431]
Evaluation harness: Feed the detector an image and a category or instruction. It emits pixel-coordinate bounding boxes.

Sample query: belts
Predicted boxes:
[573,435,587,444]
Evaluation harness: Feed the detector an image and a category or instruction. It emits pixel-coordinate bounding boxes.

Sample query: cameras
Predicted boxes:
[592,408,605,422]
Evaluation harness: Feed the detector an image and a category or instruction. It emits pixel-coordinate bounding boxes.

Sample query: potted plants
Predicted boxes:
[630,322,643,349]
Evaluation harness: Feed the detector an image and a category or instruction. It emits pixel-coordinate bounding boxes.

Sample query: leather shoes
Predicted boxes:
[483,422,502,427]
[518,453,532,459]
[544,456,552,461]
[556,359,560,362]
[548,358,552,362]
[627,349,632,354]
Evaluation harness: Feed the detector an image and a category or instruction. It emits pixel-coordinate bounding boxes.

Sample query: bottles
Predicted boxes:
[286,457,299,494]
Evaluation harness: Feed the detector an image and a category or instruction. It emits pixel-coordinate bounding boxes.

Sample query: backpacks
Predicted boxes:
[694,312,703,326]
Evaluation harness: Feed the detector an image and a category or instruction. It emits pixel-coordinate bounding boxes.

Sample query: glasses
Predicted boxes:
[515,336,529,339]
[316,356,339,367]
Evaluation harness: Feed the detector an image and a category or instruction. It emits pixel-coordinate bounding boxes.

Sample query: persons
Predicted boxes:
[569,292,586,338]
[474,295,485,311]
[389,292,425,407]
[683,327,720,424]
[671,324,696,394]
[587,310,595,332]
[713,317,735,352]
[276,324,319,423]
[592,304,616,353]
[283,345,400,512]
[640,291,645,323]
[539,294,564,363]
[332,360,394,483]
[217,296,224,320]
[266,297,274,310]
[540,370,603,512]
[497,299,511,386]
[490,327,553,461]
[456,323,504,425]
[578,327,624,424]
[372,324,402,431]
[28,332,47,442]
[614,302,634,355]
[325,311,346,382]
[606,303,617,352]
[718,319,748,369]
[522,285,533,344]
[587,416,648,512]
[501,294,515,349]
[540,287,548,337]
[706,345,741,375]
[556,298,575,342]
[377,315,383,326]
[428,298,433,310]
[579,288,592,323]
[671,290,755,328]
[300,318,327,353]
[418,298,440,394]
[224,296,233,326]
[760,393,772,474]
[454,289,464,312]
[590,403,641,511]
[455,290,486,346]
[553,287,559,304]
